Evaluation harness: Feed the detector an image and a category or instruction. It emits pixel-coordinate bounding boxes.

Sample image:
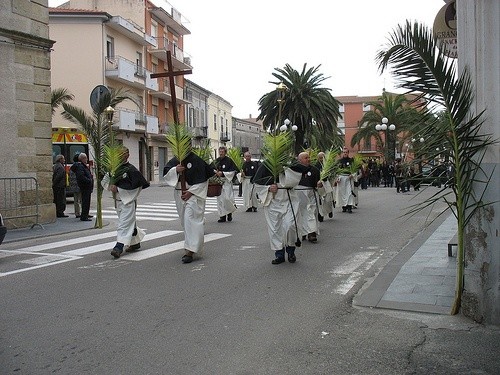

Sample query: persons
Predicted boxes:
[160,136,216,264]
[209,146,240,223]
[69,153,93,221]
[52,154,69,218]
[360,158,420,193]
[253,136,362,265]
[101,146,149,258]
[240,152,261,213]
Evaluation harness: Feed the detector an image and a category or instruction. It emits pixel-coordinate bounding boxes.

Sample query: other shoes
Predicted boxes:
[246,208,252,212]
[57,215,69,217]
[182,255,193,264]
[271,256,286,264]
[227,213,232,221]
[343,207,347,212]
[307,232,317,241]
[217,215,226,222]
[347,208,352,213]
[254,208,257,212]
[329,212,333,218]
[288,253,296,263]
[87,215,93,218]
[111,244,124,258]
[125,244,141,251]
[80,218,92,221]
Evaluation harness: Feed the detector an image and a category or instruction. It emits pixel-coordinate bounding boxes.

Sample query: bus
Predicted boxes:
[52,126,95,196]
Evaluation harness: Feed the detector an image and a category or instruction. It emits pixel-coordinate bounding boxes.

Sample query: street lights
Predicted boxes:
[275,80,287,134]
[104,105,116,146]
[376,116,396,165]
